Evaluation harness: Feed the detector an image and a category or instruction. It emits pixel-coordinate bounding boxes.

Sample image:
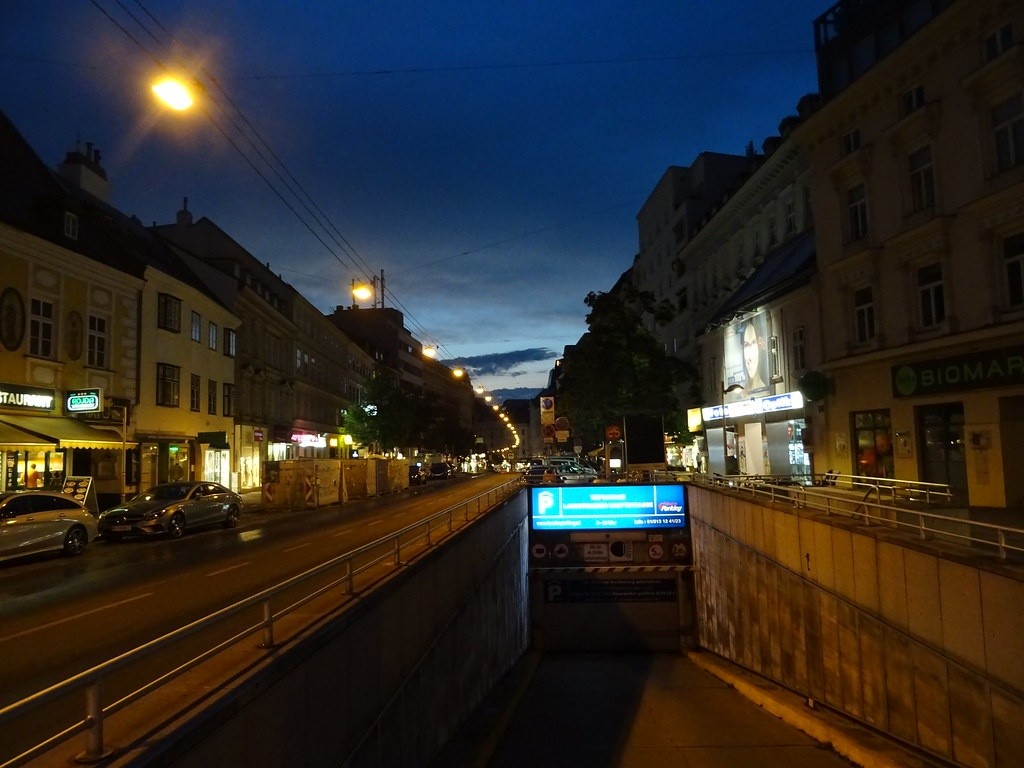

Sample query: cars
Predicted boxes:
[0,492,98,561]
[523,456,600,484]
[428,462,452,481]
[409,465,426,485]
[98,480,243,542]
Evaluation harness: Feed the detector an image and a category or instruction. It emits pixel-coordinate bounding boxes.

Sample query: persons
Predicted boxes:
[696,453,702,473]
[24,464,41,488]
[787,423,794,440]
[737,309,770,401]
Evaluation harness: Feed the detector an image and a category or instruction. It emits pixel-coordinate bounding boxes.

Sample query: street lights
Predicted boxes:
[720,378,746,477]
